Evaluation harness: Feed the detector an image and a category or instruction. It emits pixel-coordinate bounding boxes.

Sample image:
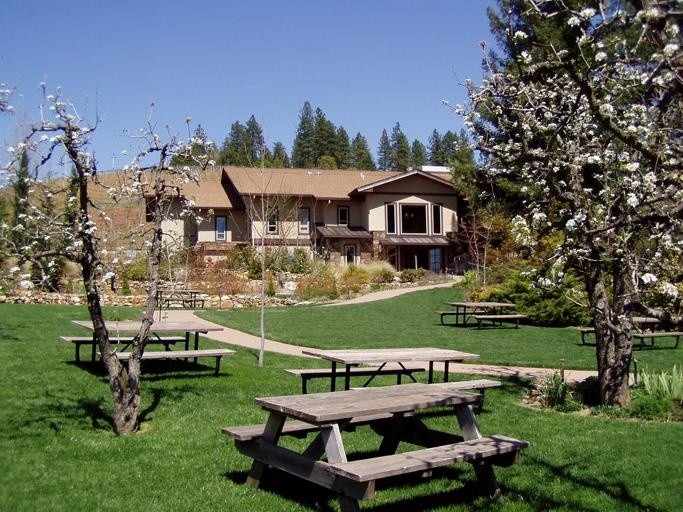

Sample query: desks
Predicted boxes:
[443,301,516,327]
[157,290,200,307]
[72,317,225,343]
[244,383,500,511]
[319,344,480,383]
[625,316,682,346]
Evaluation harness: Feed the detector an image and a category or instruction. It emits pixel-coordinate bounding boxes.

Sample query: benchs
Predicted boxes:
[577,328,596,345]
[161,298,193,308]
[99,348,235,379]
[284,365,427,393]
[631,332,683,350]
[194,298,207,308]
[60,334,186,359]
